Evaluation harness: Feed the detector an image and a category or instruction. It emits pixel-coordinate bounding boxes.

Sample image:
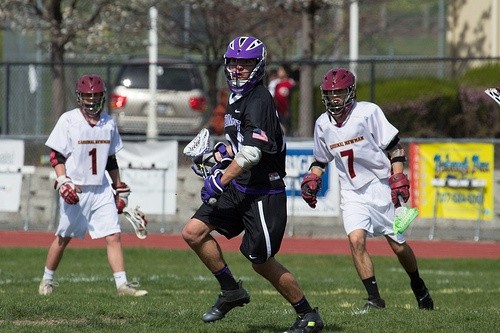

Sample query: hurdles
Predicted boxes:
[47,170,60,232]
[0,163,36,232]
[284,170,301,237]
[117,161,169,234]
[427,178,487,242]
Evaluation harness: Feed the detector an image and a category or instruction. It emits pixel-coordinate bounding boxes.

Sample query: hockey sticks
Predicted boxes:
[122,205,149,240]
[392,194,418,235]
[484,86,500,105]
[182,127,218,207]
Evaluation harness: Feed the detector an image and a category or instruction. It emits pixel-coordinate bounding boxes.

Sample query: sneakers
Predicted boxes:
[38,278,59,296]
[362,295,385,309]
[285,305,324,333]
[409,278,434,310]
[116,280,148,297]
[201,280,251,323]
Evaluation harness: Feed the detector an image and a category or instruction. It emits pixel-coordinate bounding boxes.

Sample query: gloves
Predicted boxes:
[110,181,130,213]
[389,173,409,207]
[301,172,322,208]
[190,149,225,180]
[54,175,82,205]
[201,171,225,207]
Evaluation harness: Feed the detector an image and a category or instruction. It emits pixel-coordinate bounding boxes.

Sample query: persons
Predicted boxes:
[39,75,149,296]
[180,36,325,332]
[268,64,297,137]
[299,68,434,309]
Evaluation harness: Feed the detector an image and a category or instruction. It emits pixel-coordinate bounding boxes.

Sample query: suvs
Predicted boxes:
[108,54,215,136]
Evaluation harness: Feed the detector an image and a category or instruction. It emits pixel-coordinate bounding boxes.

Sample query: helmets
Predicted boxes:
[320,68,355,90]
[76,74,106,94]
[222,35,266,93]
